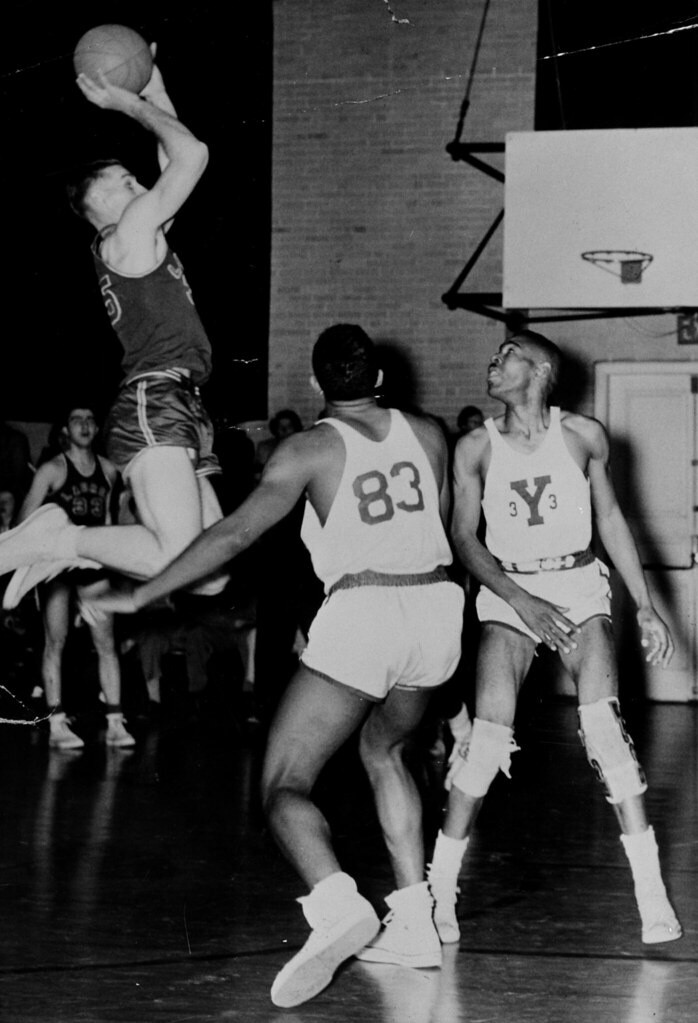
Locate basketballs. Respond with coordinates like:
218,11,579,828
74,20,153,93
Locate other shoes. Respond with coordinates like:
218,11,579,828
136,701,168,720
241,691,262,725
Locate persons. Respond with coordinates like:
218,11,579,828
0,40,231,610
19,405,136,750
425,330,684,944
458,406,483,432
1,408,327,731
80,323,465,1008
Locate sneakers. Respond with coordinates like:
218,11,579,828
353,882,444,968
0,502,83,576
2,557,102,610
106,713,135,745
47,712,85,748
271,872,380,1008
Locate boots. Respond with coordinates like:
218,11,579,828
425,830,471,943
618,825,682,943
